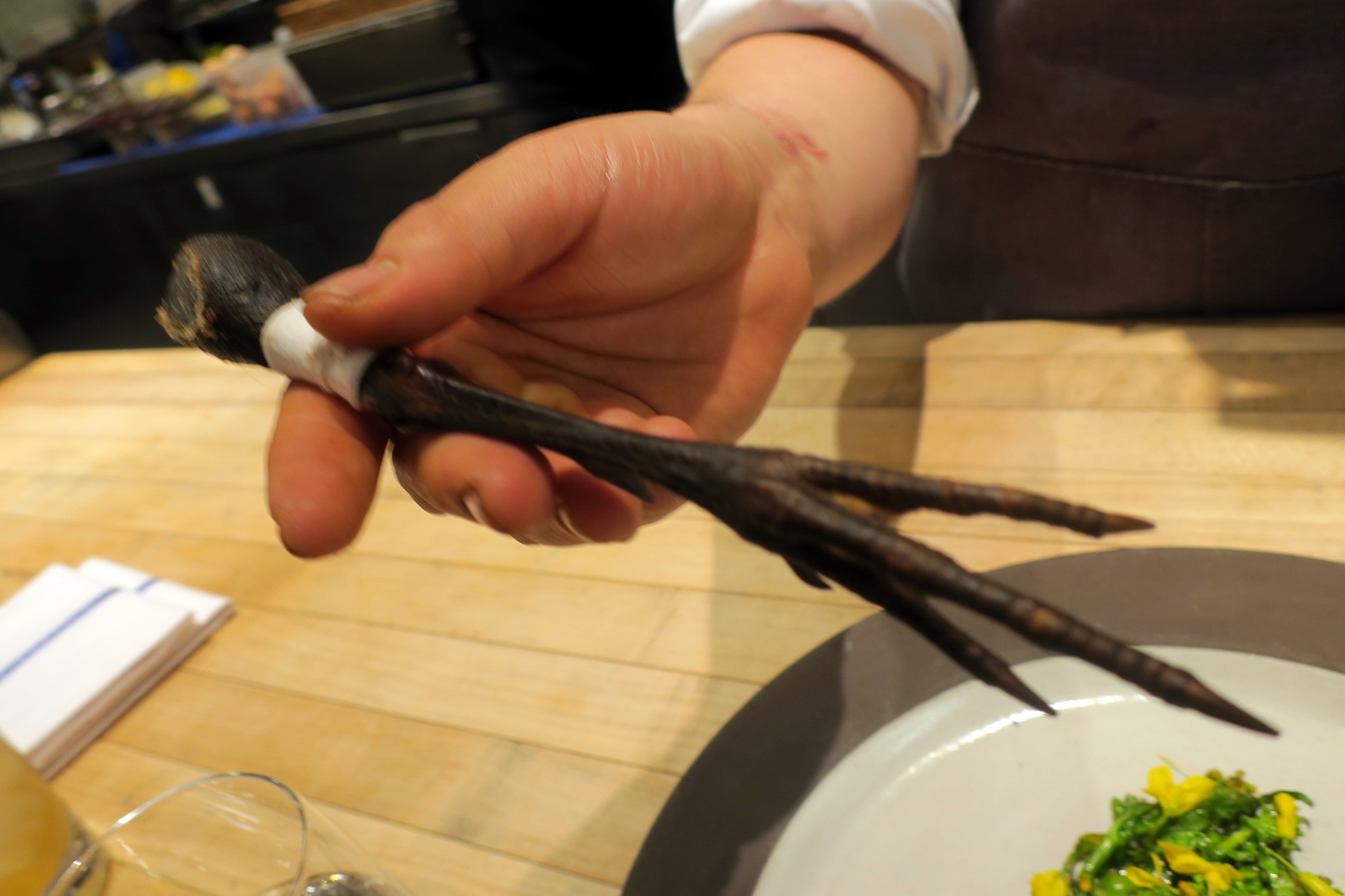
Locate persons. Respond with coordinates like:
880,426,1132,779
266,0,1345,558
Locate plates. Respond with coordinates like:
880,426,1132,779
621,547,1345,896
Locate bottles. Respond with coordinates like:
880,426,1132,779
39,92,75,122
10,77,37,113
81,67,123,100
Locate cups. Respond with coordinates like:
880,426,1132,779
0,735,108,896
45,772,410,896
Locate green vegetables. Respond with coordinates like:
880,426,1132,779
1031,766,1344,896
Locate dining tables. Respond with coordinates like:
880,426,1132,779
0,322,1345,896
